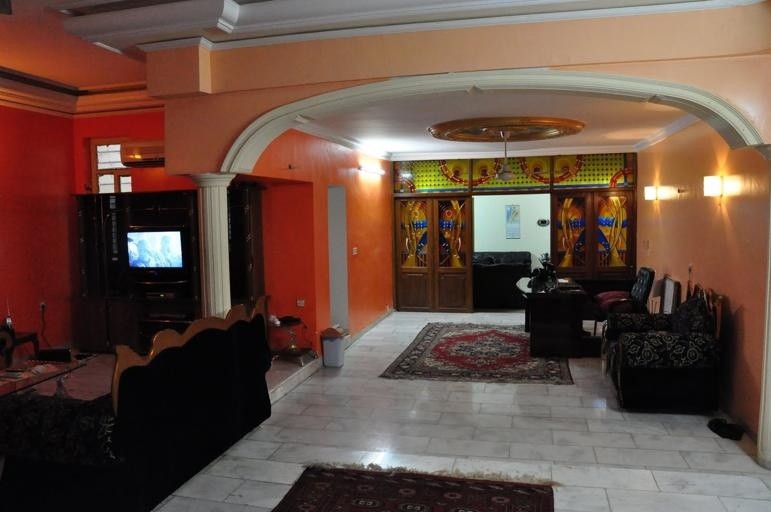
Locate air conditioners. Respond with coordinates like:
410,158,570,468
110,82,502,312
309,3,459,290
118,139,164,168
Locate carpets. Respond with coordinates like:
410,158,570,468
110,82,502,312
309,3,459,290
272,459,557,512
376,322,575,386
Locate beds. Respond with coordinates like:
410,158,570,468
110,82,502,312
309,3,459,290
0,293,275,512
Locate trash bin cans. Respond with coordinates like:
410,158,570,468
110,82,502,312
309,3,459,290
320,324,346,368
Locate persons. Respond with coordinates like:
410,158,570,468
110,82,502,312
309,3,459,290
128,236,176,267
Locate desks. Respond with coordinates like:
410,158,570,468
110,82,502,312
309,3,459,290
514,276,587,359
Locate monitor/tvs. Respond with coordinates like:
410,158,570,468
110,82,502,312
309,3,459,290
124,224,184,281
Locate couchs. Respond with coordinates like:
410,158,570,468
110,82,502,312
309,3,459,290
472,250,532,311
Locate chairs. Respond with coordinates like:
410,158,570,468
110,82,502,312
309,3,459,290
591,266,722,414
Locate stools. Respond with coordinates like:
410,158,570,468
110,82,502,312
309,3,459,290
271,316,318,366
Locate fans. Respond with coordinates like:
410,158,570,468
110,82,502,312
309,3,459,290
475,129,551,184
